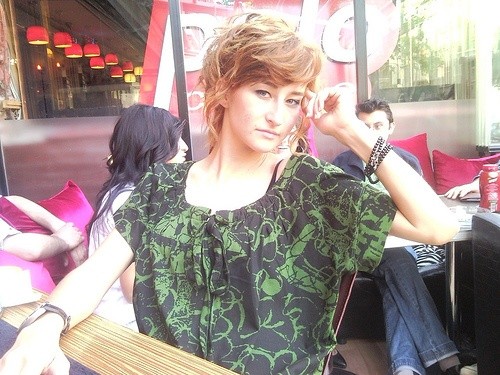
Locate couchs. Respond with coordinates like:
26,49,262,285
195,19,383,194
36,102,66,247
0,98,478,343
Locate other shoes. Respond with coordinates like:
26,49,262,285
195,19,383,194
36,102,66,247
333,350,347,368
446,363,464,375
331,369,355,375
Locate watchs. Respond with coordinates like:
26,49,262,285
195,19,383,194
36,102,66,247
16,301,71,338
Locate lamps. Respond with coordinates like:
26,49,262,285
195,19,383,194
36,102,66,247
54,10,143,83
25,0,49,46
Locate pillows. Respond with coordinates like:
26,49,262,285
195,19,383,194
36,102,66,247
390,132,433,186
433,150,500,195
37,179,94,269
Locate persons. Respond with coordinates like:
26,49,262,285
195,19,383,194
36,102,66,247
1,13,460,375
445,170,481,200
83,103,189,334
0,192,87,269
331,99,464,375
292,136,349,369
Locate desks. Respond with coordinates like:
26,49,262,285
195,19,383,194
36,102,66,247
384,203,493,375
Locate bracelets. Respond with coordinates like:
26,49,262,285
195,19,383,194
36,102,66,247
364,137,394,183
